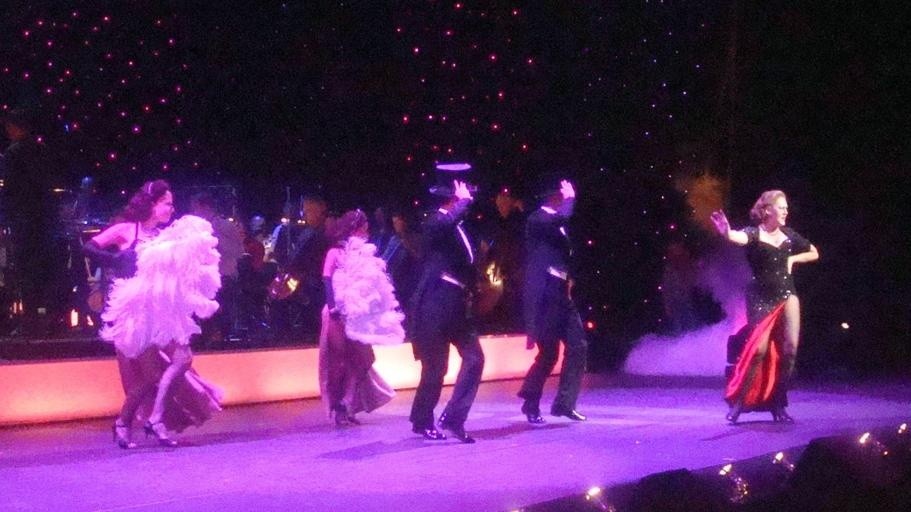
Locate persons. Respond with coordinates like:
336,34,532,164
663,240,705,337
517,177,589,424
403,160,485,443
316,208,396,426
1,110,532,356
710,189,819,424
83,179,223,449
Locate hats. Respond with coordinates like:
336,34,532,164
427,161,480,199
532,172,563,200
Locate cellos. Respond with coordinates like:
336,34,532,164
472,195,522,316
269,210,328,306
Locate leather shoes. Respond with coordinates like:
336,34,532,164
724,410,742,426
436,409,477,444
520,399,548,424
411,421,448,441
328,401,351,427
549,407,587,422
769,408,794,425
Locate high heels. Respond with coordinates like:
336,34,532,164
348,415,362,425
111,420,139,450
142,418,178,448
